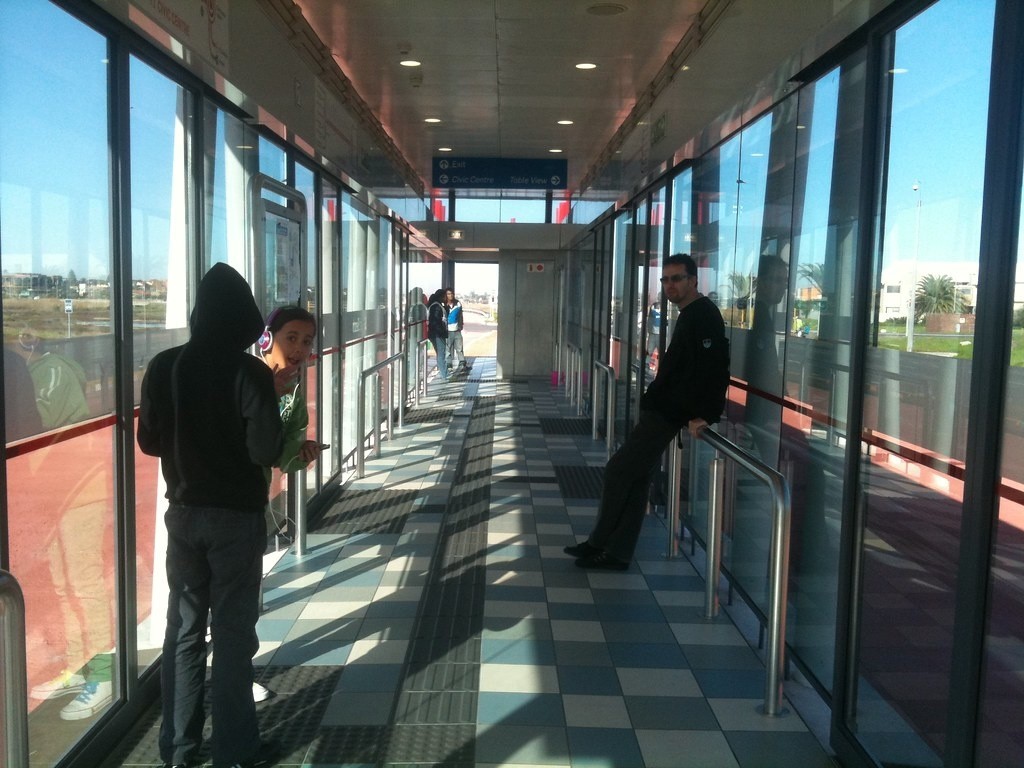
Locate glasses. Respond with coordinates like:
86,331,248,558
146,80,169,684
660,274,694,284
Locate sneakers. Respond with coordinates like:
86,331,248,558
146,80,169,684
60,680,112,720
30,669,87,699
564,541,604,557
252,682,270,702
230,739,281,768
578,553,629,571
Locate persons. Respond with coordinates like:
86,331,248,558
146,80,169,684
244,304,330,703
3,311,126,719
644,292,668,373
428,288,473,381
136,262,281,768
726,255,826,575
562,254,731,573
405,286,429,343
708,292,719,305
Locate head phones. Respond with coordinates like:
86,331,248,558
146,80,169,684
258,307,296,351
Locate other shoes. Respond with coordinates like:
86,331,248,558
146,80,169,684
448,368,454,374
459,363,472,372
